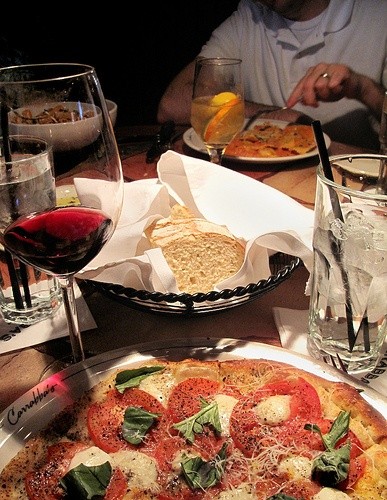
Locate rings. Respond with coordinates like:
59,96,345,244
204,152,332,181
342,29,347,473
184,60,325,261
319,73,331,79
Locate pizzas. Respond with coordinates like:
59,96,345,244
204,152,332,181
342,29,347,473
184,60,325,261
5,359,387,496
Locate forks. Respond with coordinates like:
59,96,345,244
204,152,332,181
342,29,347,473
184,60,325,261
152,121,190,164
241,95,305,134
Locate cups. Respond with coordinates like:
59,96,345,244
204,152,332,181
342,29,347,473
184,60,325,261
306,152,387,376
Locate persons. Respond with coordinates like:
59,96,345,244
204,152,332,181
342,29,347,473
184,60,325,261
159,0,387,151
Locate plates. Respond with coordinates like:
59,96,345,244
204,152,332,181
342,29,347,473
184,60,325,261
1,337,384,499
184,117,331,163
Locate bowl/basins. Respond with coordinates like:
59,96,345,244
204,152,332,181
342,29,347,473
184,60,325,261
4,100,121,152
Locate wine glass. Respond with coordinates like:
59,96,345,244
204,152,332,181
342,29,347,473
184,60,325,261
193,56,244,163
0,136,58,325
0,65,124,371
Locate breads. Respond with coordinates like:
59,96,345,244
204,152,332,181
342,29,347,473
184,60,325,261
143,202,246,295
